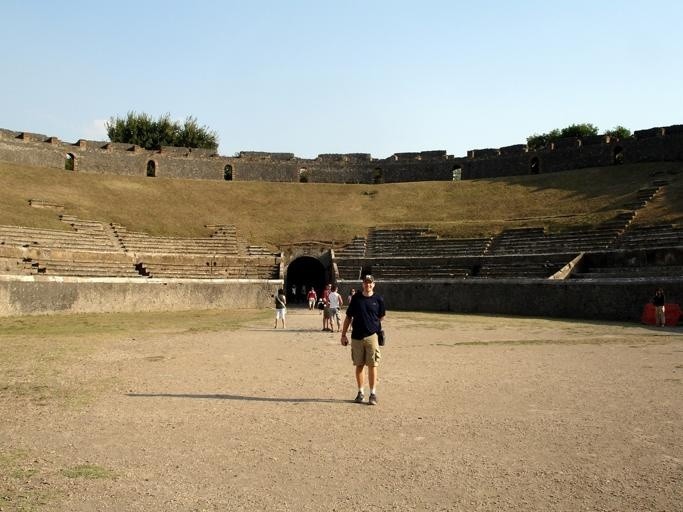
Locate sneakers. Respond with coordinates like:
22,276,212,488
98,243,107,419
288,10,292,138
331,329,334,332
369,393,377,405
275,326,285,328
355,392,365,401
337,329,340,332
323,328,331,331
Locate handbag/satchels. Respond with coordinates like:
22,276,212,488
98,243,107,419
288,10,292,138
378,322,386,347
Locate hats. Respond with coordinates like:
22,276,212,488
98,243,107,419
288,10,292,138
363,275,374,283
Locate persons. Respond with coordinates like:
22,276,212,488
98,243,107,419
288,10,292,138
275,289,286,328
653,288,665,328
341,274,385,405
347,288,356,331
291,283,343,332
